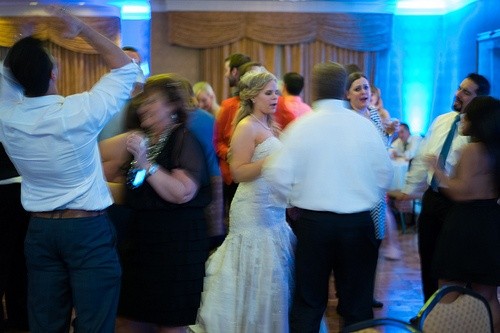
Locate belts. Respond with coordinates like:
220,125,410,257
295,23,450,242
30,210,107,218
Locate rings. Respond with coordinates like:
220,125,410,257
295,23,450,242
56,7,65,18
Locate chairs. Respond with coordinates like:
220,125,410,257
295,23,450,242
390,158,416,233
343,283,494,333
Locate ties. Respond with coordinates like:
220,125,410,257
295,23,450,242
430,114,460,191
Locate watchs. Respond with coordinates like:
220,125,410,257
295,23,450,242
144,163,161,180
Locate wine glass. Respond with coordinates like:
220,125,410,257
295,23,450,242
383,118,397,149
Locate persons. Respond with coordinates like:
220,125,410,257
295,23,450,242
214,62,296,239
193,81,220,114
98,73,213,333
368,84,398,239
196,66,297,333
0,6,146,331
263,61,407,333
399,73,492,324
422,95,500,332
282,71,312,117
224,53,251,98
184,81,225,249
98,47,142,133
392,122,419,160
344,62,416,261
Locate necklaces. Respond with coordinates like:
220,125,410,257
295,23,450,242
250,112,273,132
